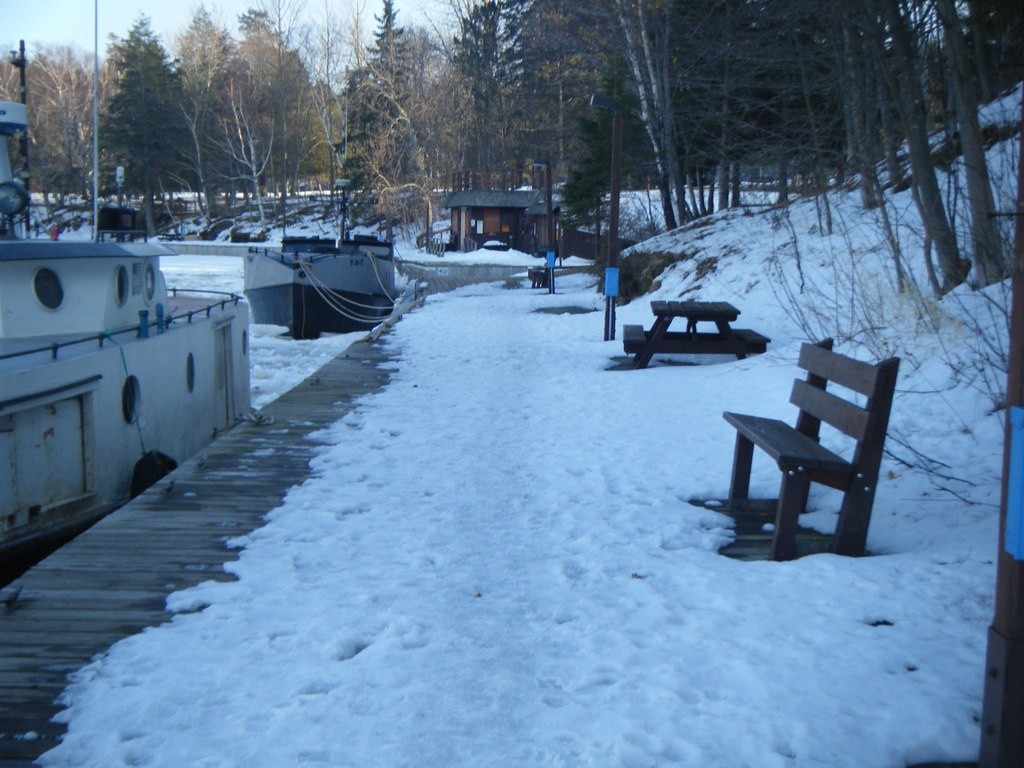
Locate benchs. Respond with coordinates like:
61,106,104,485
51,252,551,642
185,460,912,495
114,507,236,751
732,328,771,354
622,324,645,354
723,336,902,560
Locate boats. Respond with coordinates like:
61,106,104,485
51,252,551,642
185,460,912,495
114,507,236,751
241,175,398,340
0,2,252,570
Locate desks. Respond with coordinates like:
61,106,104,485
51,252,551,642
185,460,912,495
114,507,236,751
630,300,747,370
